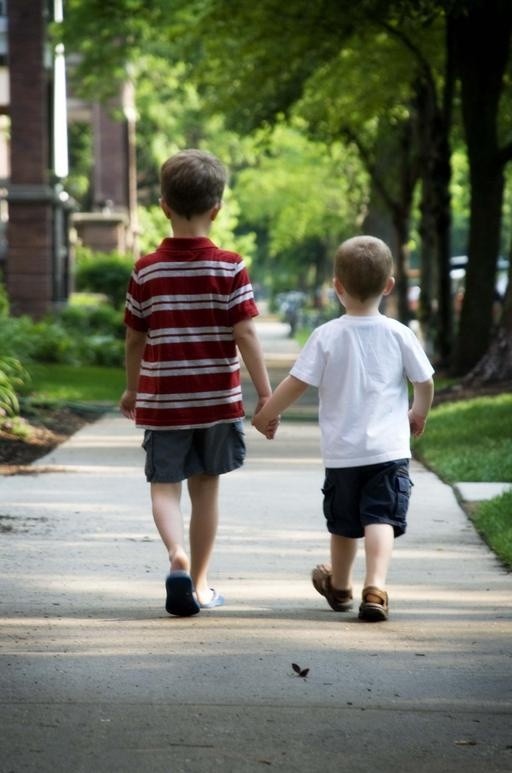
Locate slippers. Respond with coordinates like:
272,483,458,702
200,587,224,608
164,569,201,615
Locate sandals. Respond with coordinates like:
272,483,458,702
357,586,390,620
311,563,354,611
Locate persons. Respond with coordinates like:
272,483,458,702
255,234,436,621
119,148,282,613
